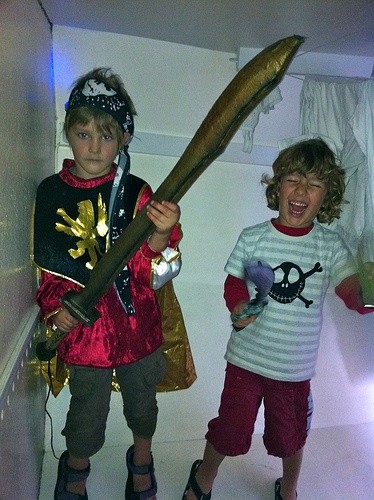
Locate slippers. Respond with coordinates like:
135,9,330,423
275,478,297,500
182,460,212,500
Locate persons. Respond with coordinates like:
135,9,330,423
29,68,197,500
183,137,374,500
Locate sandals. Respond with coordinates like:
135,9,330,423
125,444,157,500
54,450,91,500
